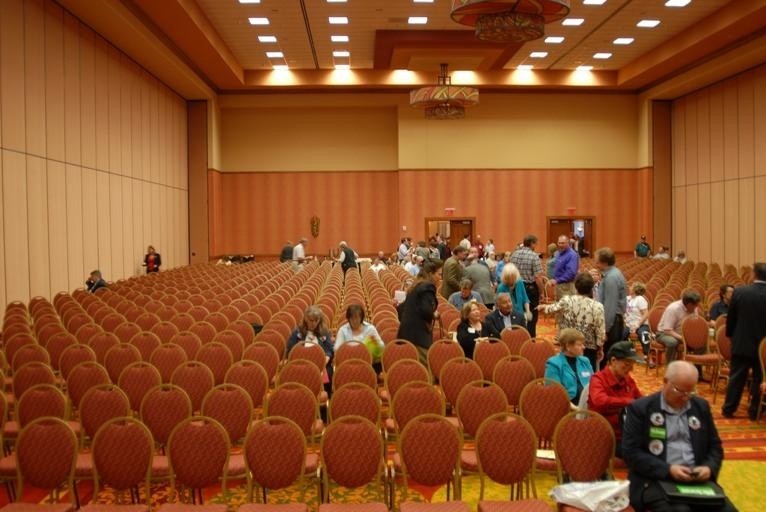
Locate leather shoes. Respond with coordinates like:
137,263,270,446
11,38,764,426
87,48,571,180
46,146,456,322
721,410,757,421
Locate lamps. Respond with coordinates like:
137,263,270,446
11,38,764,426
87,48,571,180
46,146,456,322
410,63,480,119
449,0,571,43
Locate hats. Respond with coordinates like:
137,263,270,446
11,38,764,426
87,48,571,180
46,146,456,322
607,340,647,366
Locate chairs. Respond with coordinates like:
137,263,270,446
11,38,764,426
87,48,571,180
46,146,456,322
0,258,766,512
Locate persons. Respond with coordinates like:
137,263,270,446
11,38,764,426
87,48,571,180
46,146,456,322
216,255,231,266
142,246,161,273
86,271,107,293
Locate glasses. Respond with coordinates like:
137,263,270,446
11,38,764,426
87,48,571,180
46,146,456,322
668,381,699,399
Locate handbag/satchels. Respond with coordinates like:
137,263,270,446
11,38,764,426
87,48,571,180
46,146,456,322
548,480,632,512
